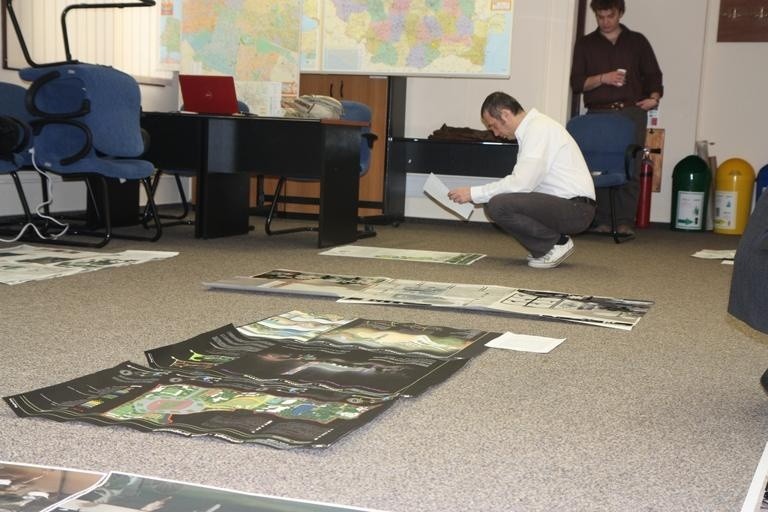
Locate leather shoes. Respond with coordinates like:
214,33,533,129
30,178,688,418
589,224,612,236
614,224,635,243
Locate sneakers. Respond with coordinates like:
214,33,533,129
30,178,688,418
525,254,535,261
527,236,576,269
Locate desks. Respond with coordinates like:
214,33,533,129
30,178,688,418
88,112,370,247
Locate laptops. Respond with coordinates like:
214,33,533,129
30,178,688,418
178,74,256,115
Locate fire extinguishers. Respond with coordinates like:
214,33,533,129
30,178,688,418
638,147,660,228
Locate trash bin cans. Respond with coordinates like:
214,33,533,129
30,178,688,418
712,159,754,237
755,165,768,201
671,155,709,232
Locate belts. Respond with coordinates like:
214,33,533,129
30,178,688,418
594,101,627,109
577,197,596,205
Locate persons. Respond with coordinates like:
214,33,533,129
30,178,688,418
570,0,664,240
447,91,596,269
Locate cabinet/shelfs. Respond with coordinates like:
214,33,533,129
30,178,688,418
395,136,519,179
192,73,406,228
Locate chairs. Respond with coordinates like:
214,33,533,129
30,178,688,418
567,112,641,243
140,100,250,227
265,101,377,239
0,0,161,249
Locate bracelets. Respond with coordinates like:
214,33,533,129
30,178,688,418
599,72,603,85
649,97,660,108
